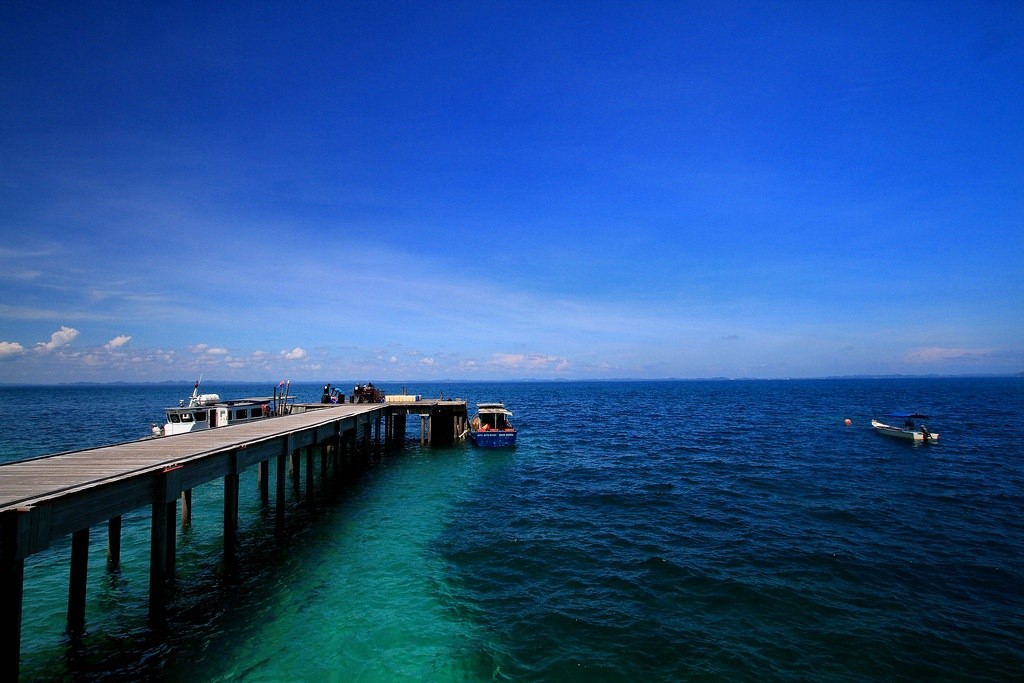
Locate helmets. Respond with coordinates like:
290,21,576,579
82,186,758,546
356,382,359,386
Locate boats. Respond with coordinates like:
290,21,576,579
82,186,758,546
872,411,939,442
145,371,298,438
471,399,518,448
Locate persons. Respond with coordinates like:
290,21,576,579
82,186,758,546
152,423,164,437
472,413,482,430
324,383,342,404
353,383,385,404
262,403,271,417
